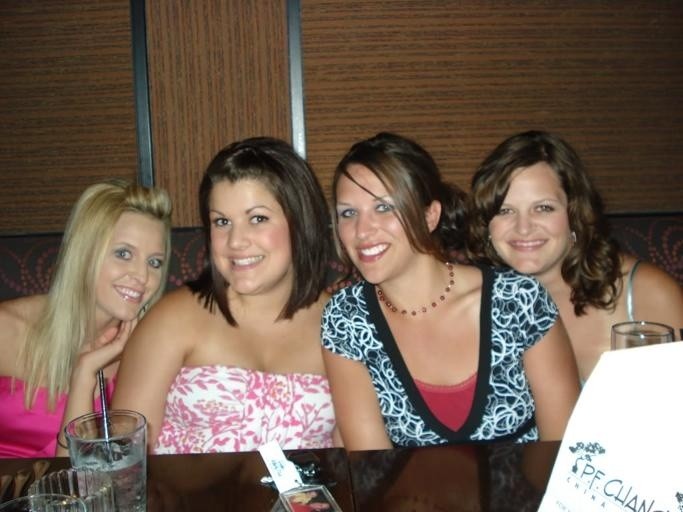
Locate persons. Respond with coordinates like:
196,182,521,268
464,128,683,382
114,133,344,455
0,178,171,459
320,131,581,452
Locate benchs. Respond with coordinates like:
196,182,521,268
1,208,683,305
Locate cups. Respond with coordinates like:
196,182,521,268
28,465,116,512
64,410,150,512
608,319,676,349
0,493,86,512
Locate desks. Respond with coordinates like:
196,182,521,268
0,434,356,511
347,437,561,510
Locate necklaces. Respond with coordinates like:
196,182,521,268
377,262,454,316
